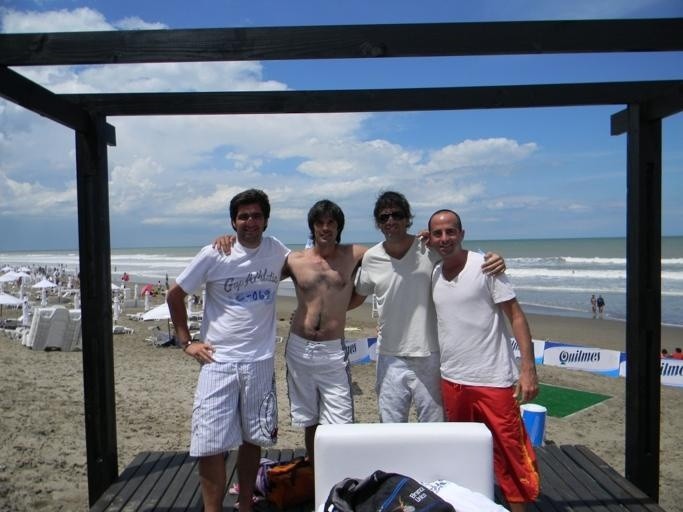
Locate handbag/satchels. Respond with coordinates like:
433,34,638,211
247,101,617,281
255,456,314,509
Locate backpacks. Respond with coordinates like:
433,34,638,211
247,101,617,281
324,470,456,512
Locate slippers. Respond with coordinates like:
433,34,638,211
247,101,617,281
229,483,260,509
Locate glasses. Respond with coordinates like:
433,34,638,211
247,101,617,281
377,211,406,224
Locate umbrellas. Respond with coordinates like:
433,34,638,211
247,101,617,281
31,278,58,303
0,291,26,305
0,271,20,282
141,301,194,340
18,266,31,272
18,272,31,299
1,266,14,271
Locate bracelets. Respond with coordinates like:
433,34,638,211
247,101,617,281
184,339,193,351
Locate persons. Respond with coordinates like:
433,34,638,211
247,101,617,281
661,349,672,358
427,209,543,504
122,272,129,289
672,348,683,359
166,188,292,512
211,197,430,465
54,268,60,283
345,189,507,422
157,280,161,291
591,295,597,314
597,294,605,314
